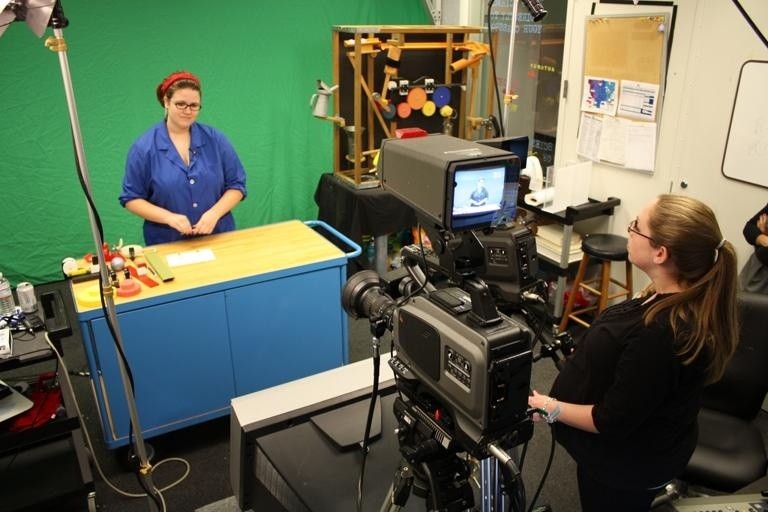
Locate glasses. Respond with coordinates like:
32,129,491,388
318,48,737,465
626,219,653,243
169,99,201,112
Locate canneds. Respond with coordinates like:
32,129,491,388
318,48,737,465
16,282,38,314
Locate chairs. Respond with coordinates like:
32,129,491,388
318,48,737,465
678,287,767,500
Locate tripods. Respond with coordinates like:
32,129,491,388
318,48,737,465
378,425,475,512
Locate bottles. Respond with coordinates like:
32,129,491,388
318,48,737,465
0,273,17,317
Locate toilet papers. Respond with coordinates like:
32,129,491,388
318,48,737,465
524,188,555,207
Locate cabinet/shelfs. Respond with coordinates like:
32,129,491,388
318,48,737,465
0,287,107,512
62,214,367,467
511,183,624,344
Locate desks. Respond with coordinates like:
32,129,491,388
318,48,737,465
312,169,423,302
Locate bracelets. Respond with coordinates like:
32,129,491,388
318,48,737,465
541,397,556,416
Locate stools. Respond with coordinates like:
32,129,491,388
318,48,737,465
554,231,635,338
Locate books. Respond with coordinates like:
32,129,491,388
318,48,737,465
535,223,584,264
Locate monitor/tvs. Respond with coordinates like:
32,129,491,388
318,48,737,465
451,160,508,219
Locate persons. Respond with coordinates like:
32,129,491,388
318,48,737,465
737,202,767,293
527,194,739,512
117,72,248,246
468,178,489,206
